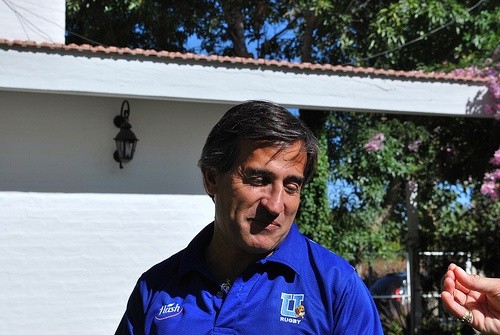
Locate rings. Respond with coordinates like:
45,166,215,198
461,310,472,326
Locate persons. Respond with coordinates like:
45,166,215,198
441,263,500,335
114,101,384,335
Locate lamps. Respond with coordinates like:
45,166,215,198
113,100,139,168
